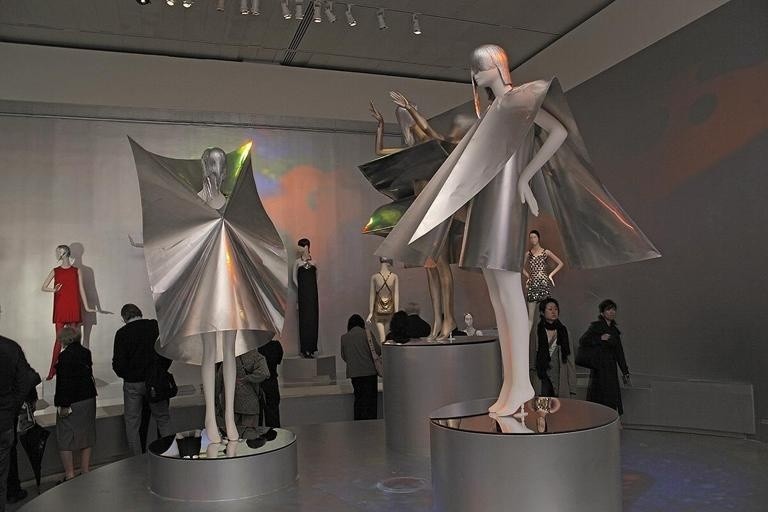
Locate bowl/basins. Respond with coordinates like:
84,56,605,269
300,255,312,269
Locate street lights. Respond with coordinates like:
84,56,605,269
145,373,178,403
574,344,602,369
365,329,383,377
258,389,268,410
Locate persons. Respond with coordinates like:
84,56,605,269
525,396,561,434
463,311,482,336
216,350,271,427
122,130,289,444
360,89,479,342
529,298,578,398
340,313,382,421
111,303,177,458
366,256,399,343
372,43,664,416
574,299,633,415
41,244,96,381
291,237,321,358
1,333,41,511
521,229,564,351
257,339,284,428
54,326,98,485
238,427,278,449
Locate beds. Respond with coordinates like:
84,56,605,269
19,422,52,494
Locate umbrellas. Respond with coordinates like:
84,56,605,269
6,490,27,504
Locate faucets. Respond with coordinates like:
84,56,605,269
56,477,66,485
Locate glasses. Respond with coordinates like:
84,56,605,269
136,0,421,35
